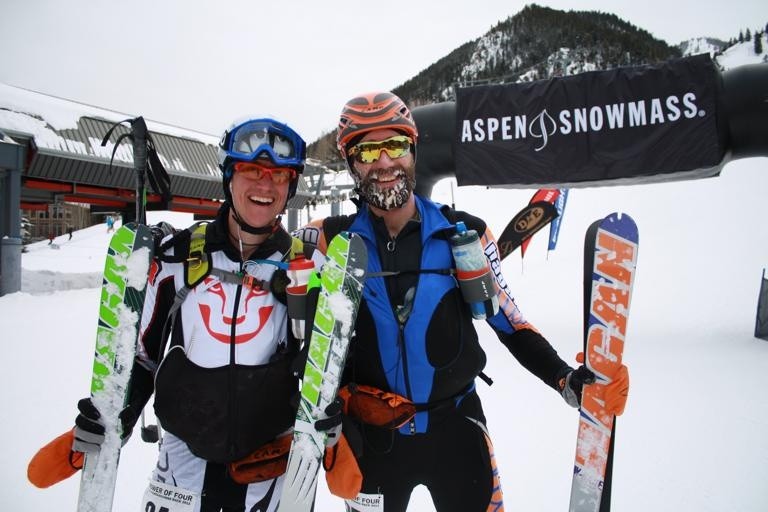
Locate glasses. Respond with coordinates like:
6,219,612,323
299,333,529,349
344,136,414,165
233,161,298,185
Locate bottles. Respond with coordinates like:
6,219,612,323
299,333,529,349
450,221,501,318
286,252,318,339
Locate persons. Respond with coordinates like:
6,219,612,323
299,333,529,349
68,110,345,512
105,214,118,234
67,223,74,241
45,230,55,246
284,90,631,511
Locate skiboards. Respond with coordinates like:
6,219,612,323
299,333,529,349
569,214,638,512
79,221,367,512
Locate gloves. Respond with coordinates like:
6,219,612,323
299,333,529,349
314,394,346,449
69,394,136,456
558,349,630,419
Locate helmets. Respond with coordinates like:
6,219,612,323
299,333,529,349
217,117,307,176
336,91,419,160
